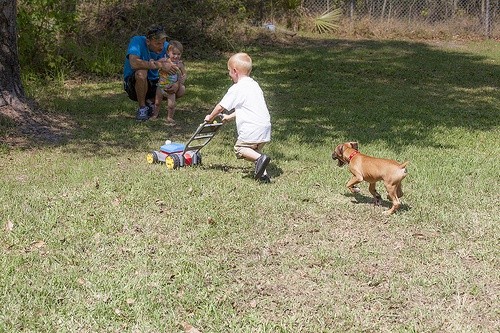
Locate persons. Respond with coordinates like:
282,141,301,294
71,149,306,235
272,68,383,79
150,41,186,122
204,52,272,184
123,24,186,121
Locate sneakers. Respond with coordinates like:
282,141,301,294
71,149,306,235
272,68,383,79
144,99,154,116
137,106,149,122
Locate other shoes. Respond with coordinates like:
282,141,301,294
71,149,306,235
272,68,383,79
252,153,270,181
242,174,271,183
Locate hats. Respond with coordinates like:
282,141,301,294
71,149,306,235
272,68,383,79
147,26,169,41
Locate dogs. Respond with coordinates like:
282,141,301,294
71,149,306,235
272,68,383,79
331,142,410,215
